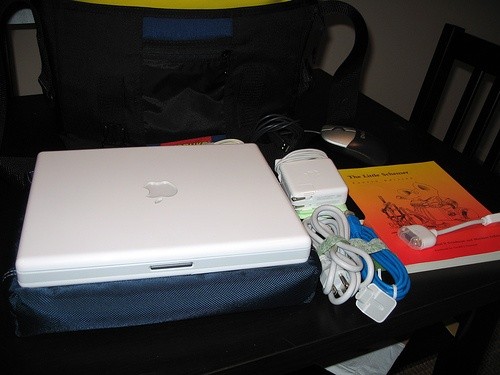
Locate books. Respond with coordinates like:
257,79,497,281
337,161,500,275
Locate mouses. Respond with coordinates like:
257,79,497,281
320,124,389,166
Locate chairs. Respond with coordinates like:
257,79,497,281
408,23,500,178
0,0,50,98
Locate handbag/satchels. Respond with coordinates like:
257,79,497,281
7,249,322,338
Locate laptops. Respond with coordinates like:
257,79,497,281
15,141,312,288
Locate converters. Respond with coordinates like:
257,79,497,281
278,157,349,211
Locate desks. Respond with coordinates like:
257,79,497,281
0,68,500,373
